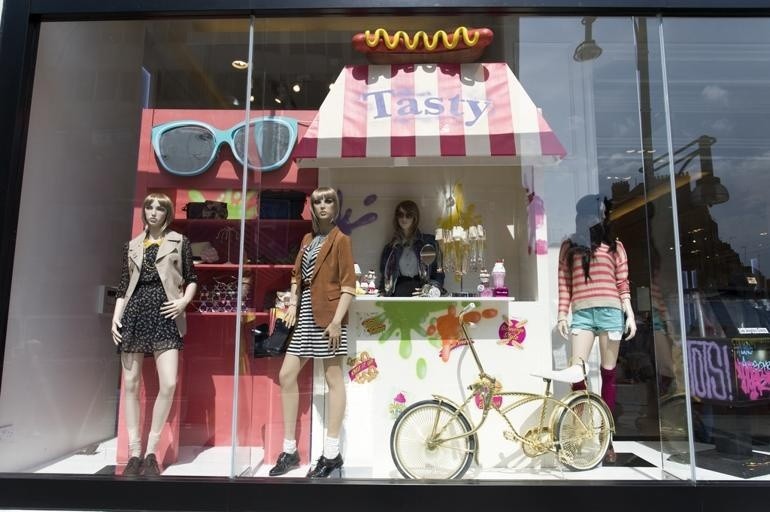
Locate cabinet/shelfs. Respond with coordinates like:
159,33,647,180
112,108,319,476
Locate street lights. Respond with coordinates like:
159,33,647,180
575,20,730,209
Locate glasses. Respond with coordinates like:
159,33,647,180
149,115,300,178
396,211,413,220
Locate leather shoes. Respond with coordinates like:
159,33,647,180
601,441,615,464
122,458,143,476
141,454,160,476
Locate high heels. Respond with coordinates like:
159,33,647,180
307,454,343,479
271,449,300,477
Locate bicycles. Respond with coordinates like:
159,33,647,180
390,302,615,480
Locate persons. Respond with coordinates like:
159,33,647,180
558,194,637,463
378,200,447,296
110,192,199,479
269,186,356,479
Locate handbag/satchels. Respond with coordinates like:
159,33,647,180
254,187,307,219
263,318,294,354
181,198,230,220
196,275,254,315
